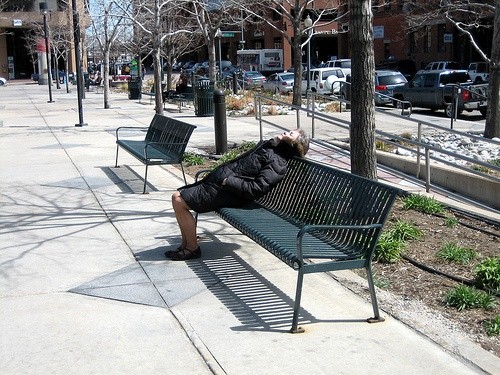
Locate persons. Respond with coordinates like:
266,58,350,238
165,129,309,261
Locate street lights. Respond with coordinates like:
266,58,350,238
216,28,223,81
305,13,315,139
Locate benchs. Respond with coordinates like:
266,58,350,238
115,115,197,195
139,83,195,113
195,155,403,333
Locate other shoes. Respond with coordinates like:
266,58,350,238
172,246,202,261
164,246,183,258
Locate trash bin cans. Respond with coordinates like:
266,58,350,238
128,80,142,100
194,81,215,117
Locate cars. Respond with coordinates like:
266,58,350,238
226,71,266,90
261,72,294,95
31,68,74,81
287,63,316,73
149,57,233,87
338,70,408,107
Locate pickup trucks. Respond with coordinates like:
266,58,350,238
392,69,489,118
467,62,491,84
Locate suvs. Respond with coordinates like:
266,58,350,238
298,67,347,96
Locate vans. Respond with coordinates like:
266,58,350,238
322,59,351,77
376,58,417,81
425,62,461,70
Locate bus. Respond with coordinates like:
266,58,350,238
237,49,284,75
